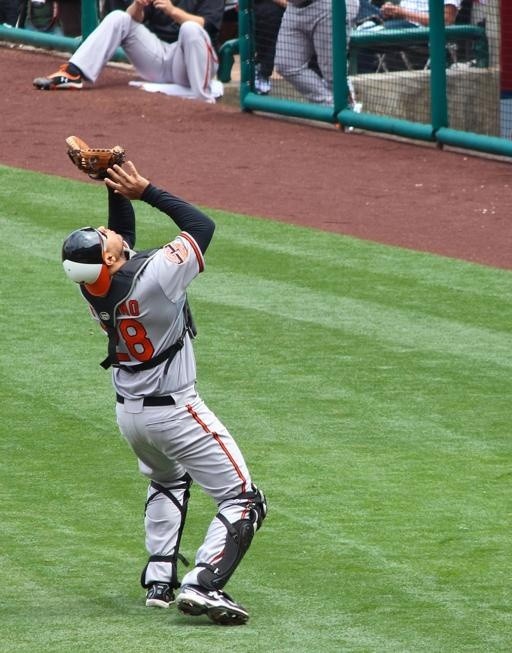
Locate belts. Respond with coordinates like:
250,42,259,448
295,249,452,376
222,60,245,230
111,392,177,407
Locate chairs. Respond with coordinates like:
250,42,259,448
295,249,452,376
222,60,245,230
371,0,474,71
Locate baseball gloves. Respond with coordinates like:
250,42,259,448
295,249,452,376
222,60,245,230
65,136,125,180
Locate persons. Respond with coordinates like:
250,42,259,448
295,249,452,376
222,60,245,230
18,1,462,133
61,136,267,626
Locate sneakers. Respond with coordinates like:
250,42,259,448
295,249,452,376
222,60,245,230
252,65,271,95
143,582,177,609
32,63,82,91
174,583,250,626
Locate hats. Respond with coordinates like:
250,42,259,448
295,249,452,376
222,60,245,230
61,225,111,297
